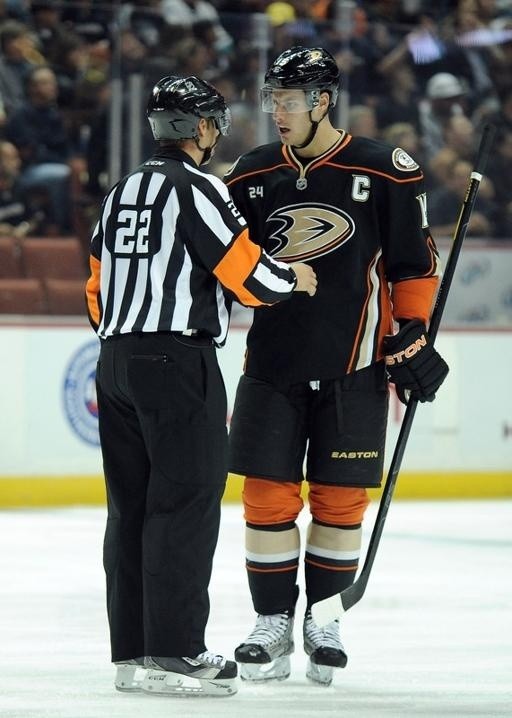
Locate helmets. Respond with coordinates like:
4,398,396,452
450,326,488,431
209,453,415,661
146,75,230,140
427,72,463,99
260,46,341,113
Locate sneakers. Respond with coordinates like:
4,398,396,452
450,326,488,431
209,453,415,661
302,610,348,668
113,651,237,679
234,610,294,662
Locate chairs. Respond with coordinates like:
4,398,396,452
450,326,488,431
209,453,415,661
0,236,48,315
19,237,92,316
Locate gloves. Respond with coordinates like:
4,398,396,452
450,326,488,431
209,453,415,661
380,317,450,404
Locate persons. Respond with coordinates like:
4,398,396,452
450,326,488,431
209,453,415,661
2,2,511,242
221,46,450,670
82,75,319,683
